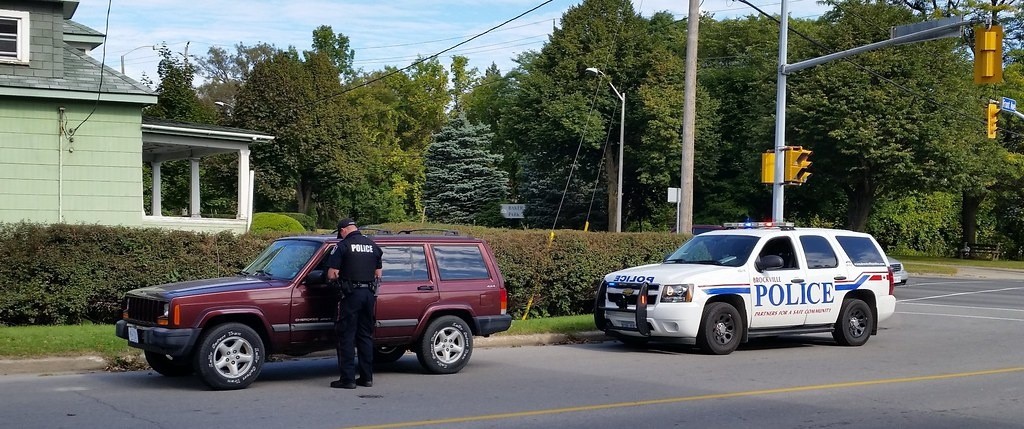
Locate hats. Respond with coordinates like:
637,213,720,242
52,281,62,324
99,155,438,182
337,218,354,239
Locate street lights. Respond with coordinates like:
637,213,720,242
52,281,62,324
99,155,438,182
586,67,626,233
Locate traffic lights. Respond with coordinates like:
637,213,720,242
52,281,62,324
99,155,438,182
802,149,813,183
988,104,1001,139
785,149,806,184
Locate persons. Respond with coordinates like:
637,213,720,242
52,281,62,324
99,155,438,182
328,219,384,389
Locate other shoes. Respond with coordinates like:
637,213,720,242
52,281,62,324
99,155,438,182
330,379,357,389
355,378,373,387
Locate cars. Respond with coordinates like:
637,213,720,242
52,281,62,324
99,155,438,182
805,250,908,287
670,225,725,235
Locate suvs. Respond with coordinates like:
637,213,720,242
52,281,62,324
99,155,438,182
115,227,513,390
593,222,896,356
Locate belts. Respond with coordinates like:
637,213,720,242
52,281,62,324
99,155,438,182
353,283,369,288
